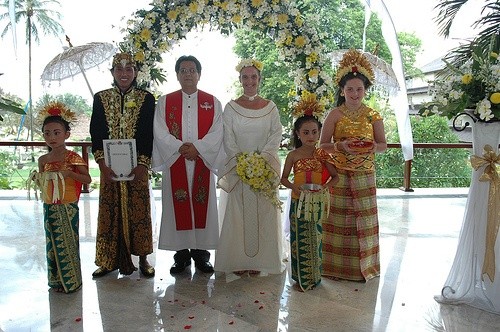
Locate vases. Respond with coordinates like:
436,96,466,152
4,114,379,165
436,122,500,314
462,109,473,126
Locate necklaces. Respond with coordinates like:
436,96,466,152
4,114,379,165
243,93,258,101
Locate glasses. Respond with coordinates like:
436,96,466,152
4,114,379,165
178,68,198,73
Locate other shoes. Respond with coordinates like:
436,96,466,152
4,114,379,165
139,260,155,278
92,266,111,277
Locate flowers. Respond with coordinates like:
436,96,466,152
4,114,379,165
337,63,372,81
236,152,283,213
420,30,500,122
110,1,335,114
36,101,77,124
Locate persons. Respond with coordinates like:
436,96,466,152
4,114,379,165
90,54,157,277
213,59,289,276
28,115,92,294
150,54,225,274
281,114,339,293
319,70,387,283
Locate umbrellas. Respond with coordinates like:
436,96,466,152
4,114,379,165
40,34,115,98
324,43,400,104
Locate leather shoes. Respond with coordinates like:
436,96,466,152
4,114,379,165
195,260,214,273
170,259,191,274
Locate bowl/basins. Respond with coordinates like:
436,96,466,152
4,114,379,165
301,184,323,193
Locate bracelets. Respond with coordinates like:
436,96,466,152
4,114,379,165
372,141,377,154
333,141,339,152
